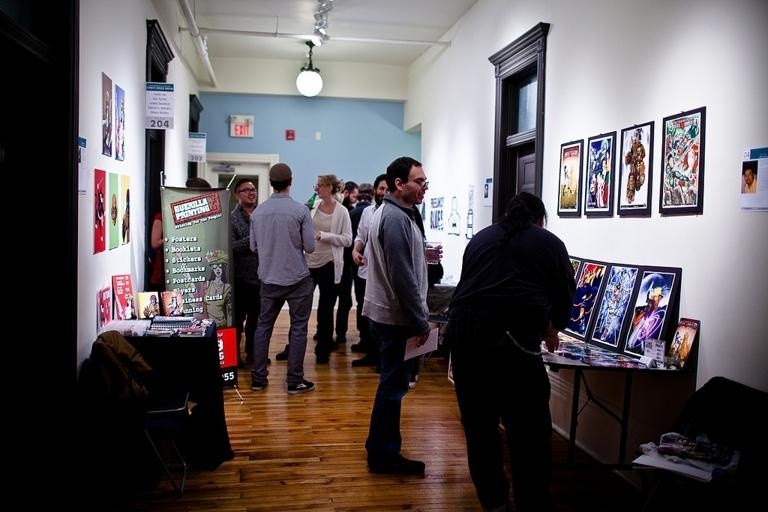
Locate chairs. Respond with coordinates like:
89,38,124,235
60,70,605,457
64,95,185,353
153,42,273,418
588,374,768,509
90,330,192,497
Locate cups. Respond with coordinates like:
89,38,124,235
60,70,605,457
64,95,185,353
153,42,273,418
424,240,442,266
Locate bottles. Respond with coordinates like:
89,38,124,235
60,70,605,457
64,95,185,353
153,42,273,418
305,192,317,208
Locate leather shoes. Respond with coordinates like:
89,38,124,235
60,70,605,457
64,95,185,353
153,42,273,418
352,358,381,366
244,354,271,369
368,453,426,475
351,341,367,352
313,332,340,364
408,374,420,389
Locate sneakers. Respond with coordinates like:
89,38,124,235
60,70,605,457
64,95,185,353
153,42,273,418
287,380,316,396
251,378,268,391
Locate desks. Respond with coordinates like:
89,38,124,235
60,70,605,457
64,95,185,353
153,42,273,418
536,347,695,478
613,454,742,511
115,318,234,472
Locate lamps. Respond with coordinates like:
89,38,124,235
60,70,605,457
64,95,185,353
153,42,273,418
294,38,325,98
310,0,334,48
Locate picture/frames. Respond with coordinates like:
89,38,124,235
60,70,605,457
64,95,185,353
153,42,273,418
560,255,684,364
557,105,708,218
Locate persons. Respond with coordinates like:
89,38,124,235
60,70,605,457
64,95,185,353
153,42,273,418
199,249,232,328
149,213,165,291
230,179,271,369
248,162,315,395
445,191,576,512
302,170,388,368
364,156,443,476
744,168,757,194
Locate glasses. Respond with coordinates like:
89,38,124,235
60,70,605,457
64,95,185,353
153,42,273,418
212,263,223,269
413,180,429,187
239,188,257,193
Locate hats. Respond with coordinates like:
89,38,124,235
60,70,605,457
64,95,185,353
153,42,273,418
269,162,292,182
209,249,229,266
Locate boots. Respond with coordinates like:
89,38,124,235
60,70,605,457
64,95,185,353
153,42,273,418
276,345,290,360
